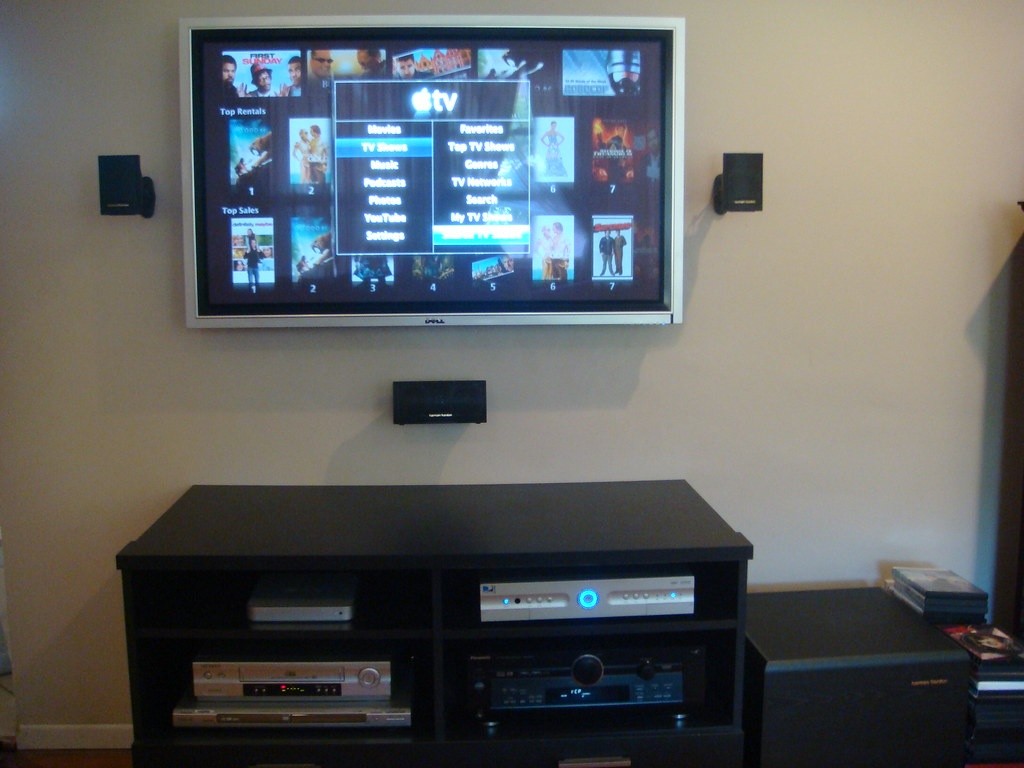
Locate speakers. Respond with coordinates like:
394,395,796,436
723,153,762,211
98,155,144,216
390,379,488,426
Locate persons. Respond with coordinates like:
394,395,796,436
357,42,386,77
238,60,290,98
307,49,335,98
541,120,569,179
293,125,328,184
287,56,302,97
502,255,514,273
232,228,274,296
599,228,627,277
537,221,570,281
222,55,239,98
393,53,434,81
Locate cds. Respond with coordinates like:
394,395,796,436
960,632,1024,653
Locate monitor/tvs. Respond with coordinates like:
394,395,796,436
178,17,683,326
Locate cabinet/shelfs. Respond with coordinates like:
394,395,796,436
115,480,753,768
